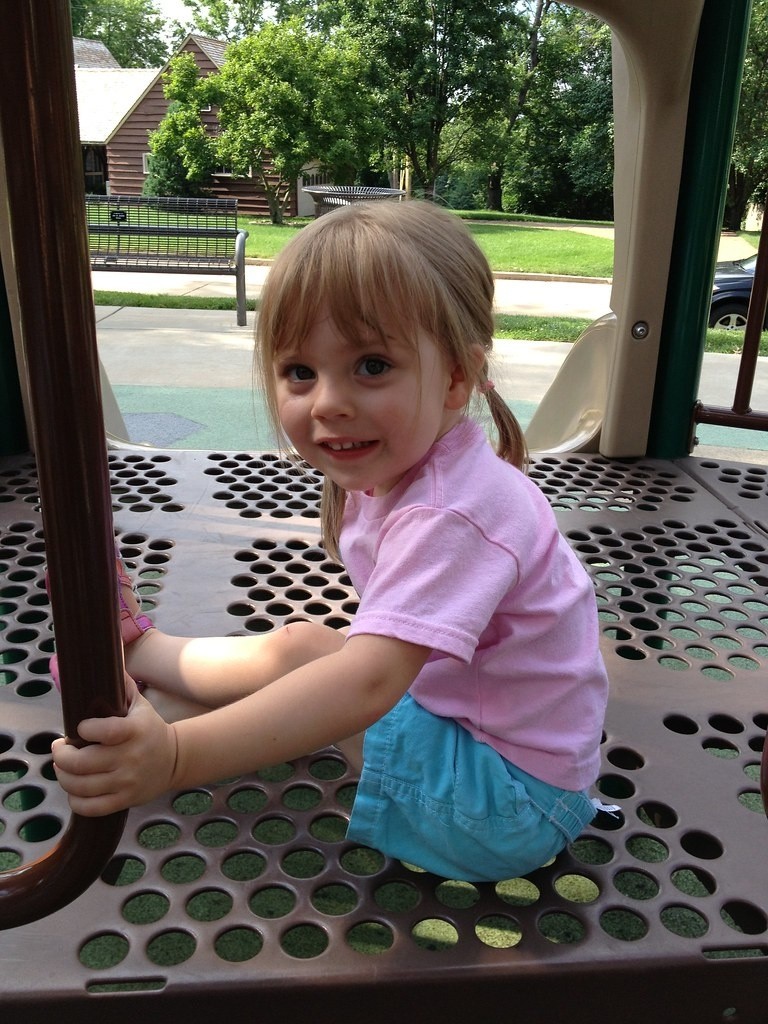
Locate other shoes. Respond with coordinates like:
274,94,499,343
44,540,155,645
49,655,61,690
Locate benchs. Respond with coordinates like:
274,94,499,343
84,193,249,327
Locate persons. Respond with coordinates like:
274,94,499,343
45,198,609,883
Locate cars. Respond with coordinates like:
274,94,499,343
707,253,768,331
